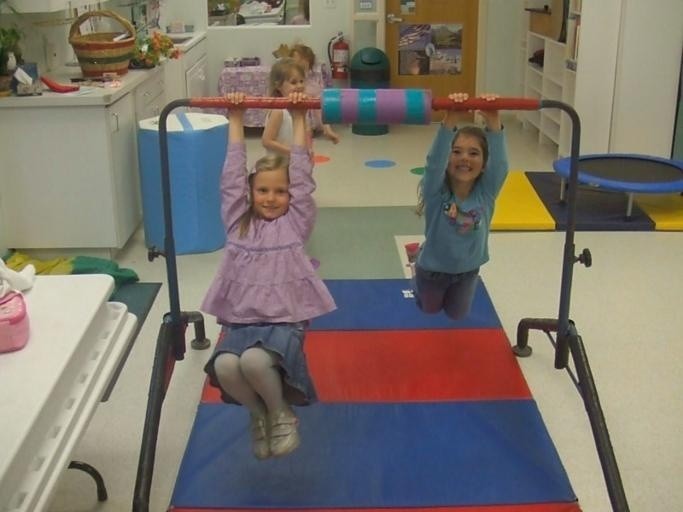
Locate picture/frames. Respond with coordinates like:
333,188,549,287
204,0,313,31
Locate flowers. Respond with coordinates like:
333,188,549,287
133,29,181,69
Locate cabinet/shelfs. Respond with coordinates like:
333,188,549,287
522,0,622,162
165,39,215,104
1,71,165,249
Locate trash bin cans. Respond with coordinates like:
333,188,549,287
350,48,390,135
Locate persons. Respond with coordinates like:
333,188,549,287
204,92,336,459
405,93,509,318
261,44,340,157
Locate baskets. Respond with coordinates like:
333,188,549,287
69,11,135,77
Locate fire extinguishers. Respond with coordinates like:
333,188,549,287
328,32,350,80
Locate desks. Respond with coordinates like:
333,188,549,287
0,269,140,509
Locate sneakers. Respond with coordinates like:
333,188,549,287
250,408,269,460
270,408,301,457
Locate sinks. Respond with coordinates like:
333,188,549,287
170,37,190,44
